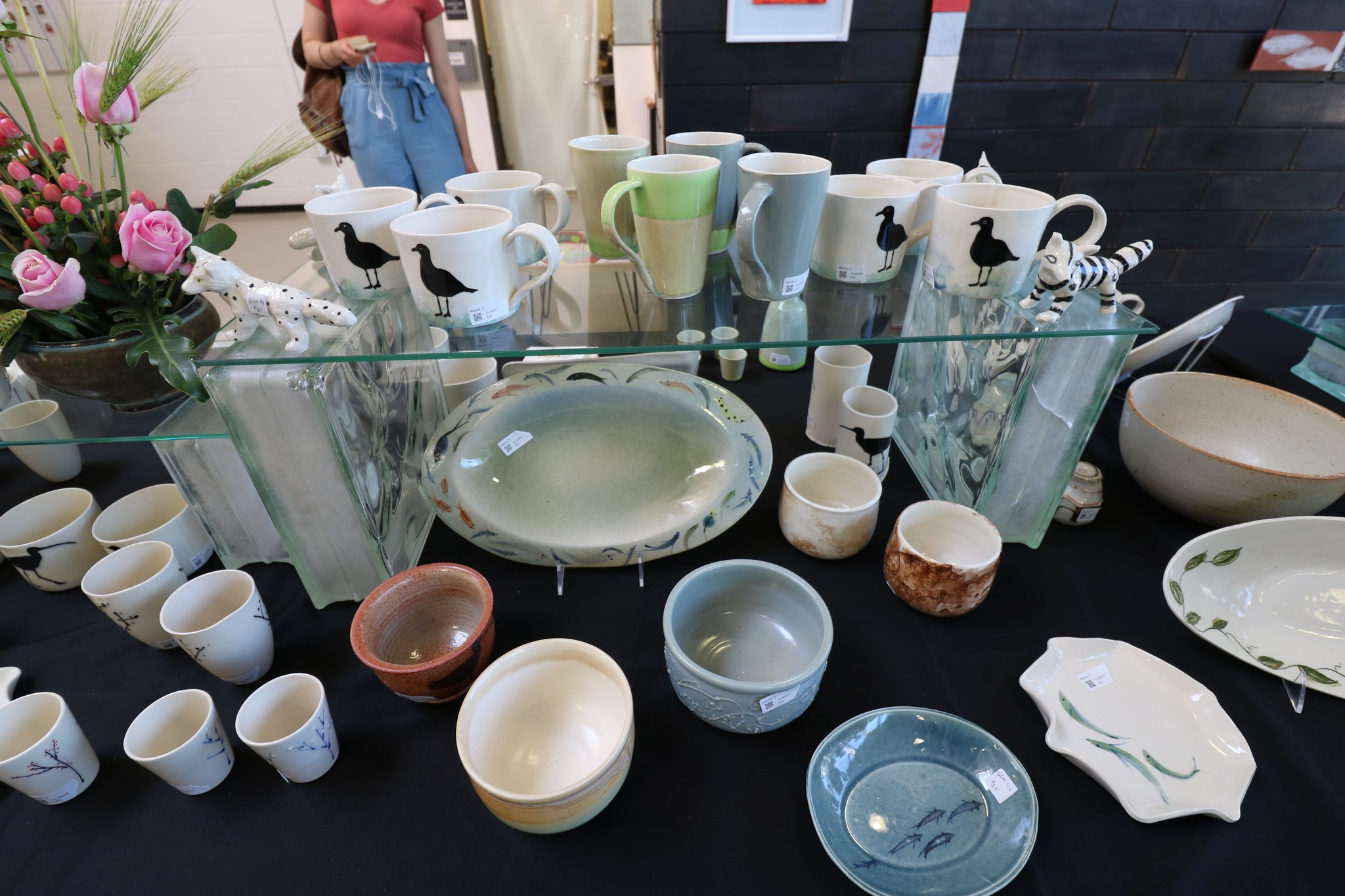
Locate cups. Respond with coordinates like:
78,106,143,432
304,186,459,299
390,204,560,328
445,171,571,267
804,343,899,484
920,182,1107,299
347,563,496,705
778,453,884,557
398,327,499,414
677,326,747,381
885,500,1003,618
0,399,341,807
1115,289,1145,315
567,131,1002,299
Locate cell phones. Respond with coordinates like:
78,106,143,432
353,42,377,54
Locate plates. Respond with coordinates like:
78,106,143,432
1120,294,1245,375
1018,636,1258,825
804,705,1039,896
418,360,774,567
1163,516,1345,699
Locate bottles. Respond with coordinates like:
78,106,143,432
758,296,808,370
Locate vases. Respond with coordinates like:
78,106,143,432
17,293,221,423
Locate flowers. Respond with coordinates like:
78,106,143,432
0,0,352,365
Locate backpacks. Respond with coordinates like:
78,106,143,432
292,0,351,158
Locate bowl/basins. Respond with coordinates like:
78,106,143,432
455,638,636,835
662,559,835,734
1118,370,1345,528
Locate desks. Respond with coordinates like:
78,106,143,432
0,330,1345,896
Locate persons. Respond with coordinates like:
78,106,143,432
301,1,480,208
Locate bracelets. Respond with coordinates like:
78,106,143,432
318,42,336,70
328,41,345,65
